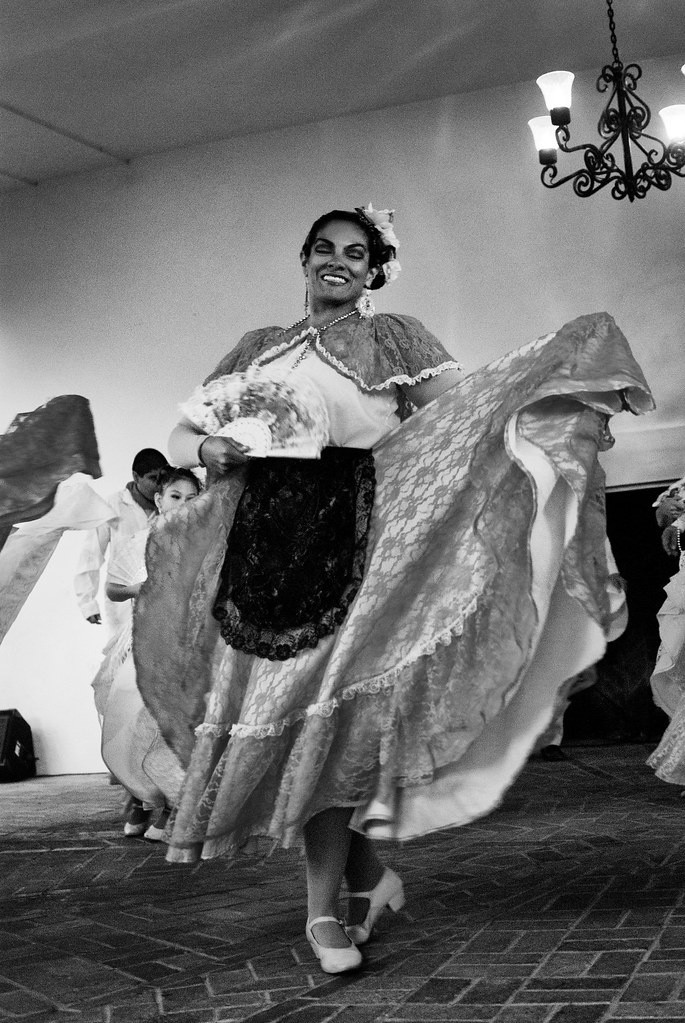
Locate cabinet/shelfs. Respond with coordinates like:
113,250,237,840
561,482,679,745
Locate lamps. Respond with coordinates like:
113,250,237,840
527,0,684,203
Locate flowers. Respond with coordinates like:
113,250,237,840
364,200,400,284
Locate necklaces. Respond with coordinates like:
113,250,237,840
278,308,361,370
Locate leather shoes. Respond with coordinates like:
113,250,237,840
339,866,405,942
124,804,152,837
304,916,362,976
145,809,172,841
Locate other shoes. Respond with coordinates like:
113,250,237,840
541,744,564,761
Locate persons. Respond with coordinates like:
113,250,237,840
644,508,685,798
130,202,655,974
102,467,206,841
78,447,170,655
